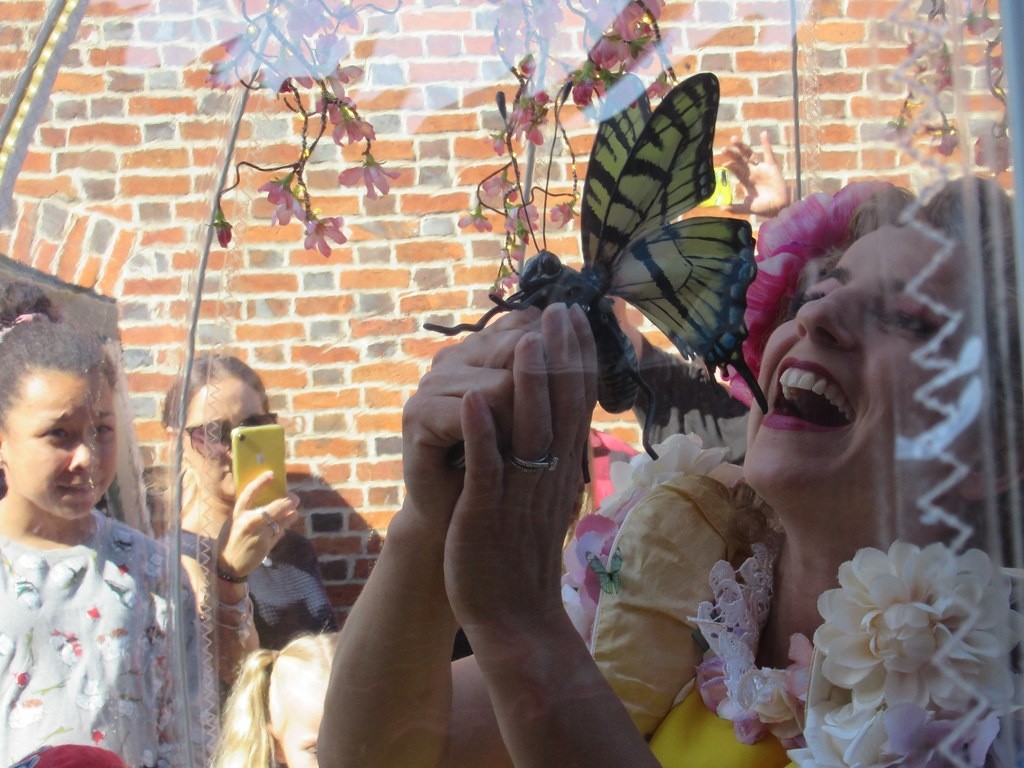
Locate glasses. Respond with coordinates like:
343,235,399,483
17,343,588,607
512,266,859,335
185,413,278,460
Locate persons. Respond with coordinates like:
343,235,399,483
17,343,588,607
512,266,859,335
0,280,220,768
162,354,339,706
211,629,341,768
318,176,1024,768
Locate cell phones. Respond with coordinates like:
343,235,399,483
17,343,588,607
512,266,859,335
231,424,286,508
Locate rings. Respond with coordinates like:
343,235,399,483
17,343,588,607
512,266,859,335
510,449,559,473
259,509,274,526
270,522,279,535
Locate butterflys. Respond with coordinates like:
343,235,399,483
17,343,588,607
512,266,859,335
422,73,769,462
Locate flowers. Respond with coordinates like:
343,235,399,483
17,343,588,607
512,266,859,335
209,0,1024,768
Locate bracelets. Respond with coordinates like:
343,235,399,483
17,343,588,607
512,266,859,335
218,568,250,583
214,584,255,647
